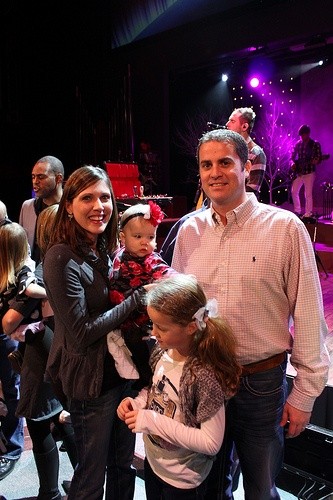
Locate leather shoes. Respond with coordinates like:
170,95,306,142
0,456,20,482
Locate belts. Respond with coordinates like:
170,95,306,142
236,353,288,378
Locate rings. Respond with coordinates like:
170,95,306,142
303,427,305,429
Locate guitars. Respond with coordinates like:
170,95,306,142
287,154,330,182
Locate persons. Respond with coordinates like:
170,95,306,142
290,124,322,221
173,129,330,500
0,156,177,500
116,275,242,499
225,107,266,205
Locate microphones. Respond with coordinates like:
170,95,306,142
207,122,227,129
